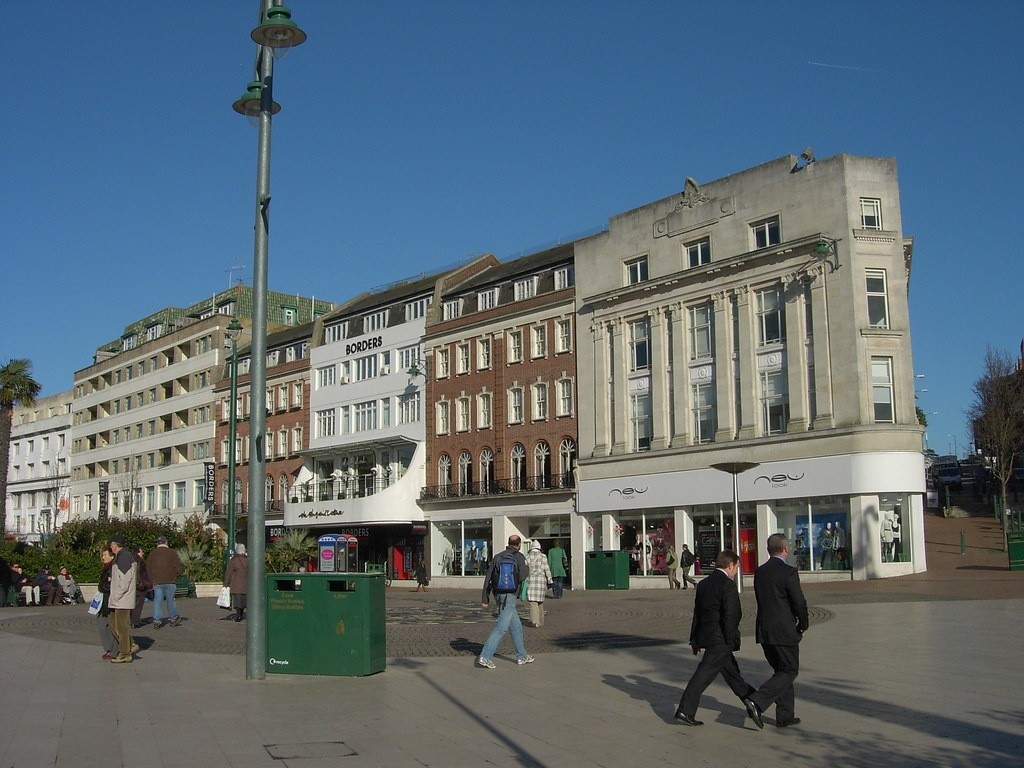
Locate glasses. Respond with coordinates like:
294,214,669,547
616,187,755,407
13,566,19,568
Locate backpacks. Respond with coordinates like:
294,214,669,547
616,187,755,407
685,552,696,566
494,552,519,593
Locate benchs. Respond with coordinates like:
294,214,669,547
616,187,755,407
172,574,196,597
6,580,77,608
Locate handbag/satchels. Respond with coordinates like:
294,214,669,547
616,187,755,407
86,589,104,616
665,551,675,566
520,579,528,602
561,548,569,572
216,585,231,608
423,578,429,586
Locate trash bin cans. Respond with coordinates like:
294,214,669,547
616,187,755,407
367,564,385,573
585,550,630,590
266,572,387,676
1007,530,1024,571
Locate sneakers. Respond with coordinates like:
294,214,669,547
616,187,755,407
110,652,133,663
130,643,140,655
516,654,536,665
477,655,497,669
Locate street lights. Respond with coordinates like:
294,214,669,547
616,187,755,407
224,312,245,565
231,0,309,682
947,434,956,455
709,461,761,595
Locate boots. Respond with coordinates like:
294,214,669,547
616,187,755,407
235,608,245,622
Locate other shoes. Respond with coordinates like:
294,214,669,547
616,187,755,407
101,651,115,660
170,619,183,627
153,620,168,629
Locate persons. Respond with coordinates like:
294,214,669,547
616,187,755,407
10,561,41,607
469,541,489,575
743,533,809,729
680,544,698,590
548,539,569,600
224,543,249,623
57,566,77,605
414,560,428,592
524,540,553,628
821,522,846,570
130,536,181,630
880,512,901,562
96,535,139,663
665,544,680,590
674,549,757,726
36,564,63,606
477,535,535,668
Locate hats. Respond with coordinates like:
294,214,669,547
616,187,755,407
157,536,167,544
59,566,65,571
109,535,126,545
41,565,49,570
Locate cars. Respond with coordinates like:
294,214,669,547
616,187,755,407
1008,468,1024,491
12,531,43,549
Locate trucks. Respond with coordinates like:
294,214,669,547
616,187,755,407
932,455,963,492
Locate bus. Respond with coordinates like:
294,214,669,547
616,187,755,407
958,459,981,489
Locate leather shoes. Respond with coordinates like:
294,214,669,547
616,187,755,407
742,697,769,731
775,717,800,726
674,710,704,726
